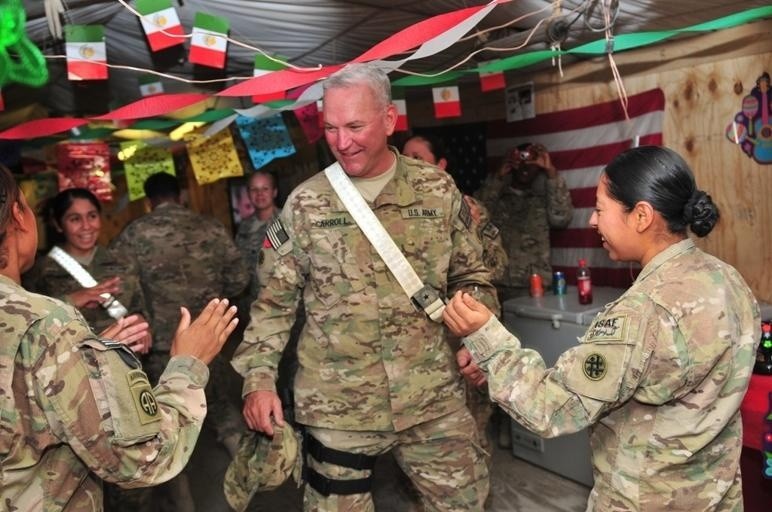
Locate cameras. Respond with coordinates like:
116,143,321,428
512,143,536,162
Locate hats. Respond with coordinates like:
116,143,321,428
220,414,299,512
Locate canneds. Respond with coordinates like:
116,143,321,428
553,271,566,296
529,273,544,297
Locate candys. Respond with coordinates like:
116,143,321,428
726,122,758,146
742,95,759,135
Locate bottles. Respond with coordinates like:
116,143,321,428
760,392,772,478
760,324,772,373
576,259,593,305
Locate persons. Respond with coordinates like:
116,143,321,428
230,61,501,512
442,145,762,512
403,135,510,458
473,142,574,449
0,165,241,512
